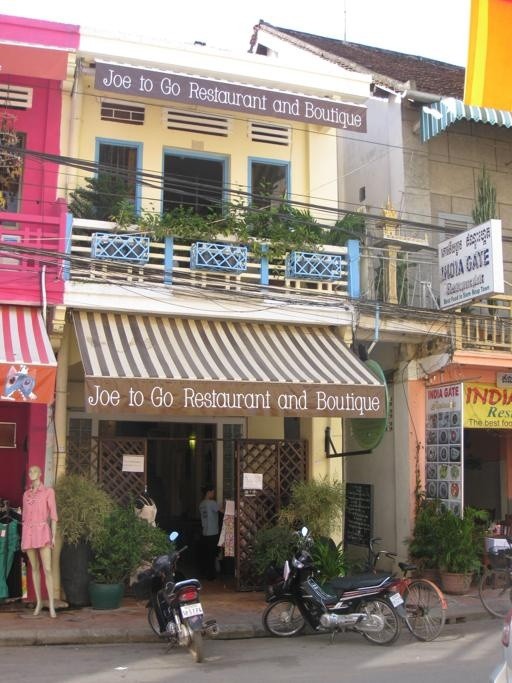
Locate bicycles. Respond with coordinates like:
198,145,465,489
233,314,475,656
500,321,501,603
360,532,452,644
477,522,511,620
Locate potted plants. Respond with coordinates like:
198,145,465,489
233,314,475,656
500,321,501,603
80,506,178,609
247,518,301,580
405,494,442,588
101,213,333,275
435,508,481,593
54,471,107,605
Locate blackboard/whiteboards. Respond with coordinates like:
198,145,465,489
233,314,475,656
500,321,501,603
344,482,374,548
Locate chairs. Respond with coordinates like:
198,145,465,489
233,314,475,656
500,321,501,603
469,504,512,590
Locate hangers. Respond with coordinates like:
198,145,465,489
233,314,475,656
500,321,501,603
0,507,23,524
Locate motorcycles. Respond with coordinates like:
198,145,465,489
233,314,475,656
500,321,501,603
133,531,221,667
261,528,405,646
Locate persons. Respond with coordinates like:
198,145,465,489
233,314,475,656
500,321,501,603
20,465,58,618
129,491,157,587
197,484,225,580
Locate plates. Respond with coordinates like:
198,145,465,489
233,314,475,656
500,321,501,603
427,412,459,514
